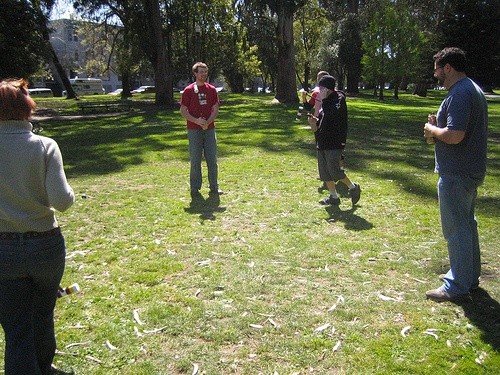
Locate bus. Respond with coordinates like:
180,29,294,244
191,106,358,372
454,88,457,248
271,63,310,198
61,77,106,97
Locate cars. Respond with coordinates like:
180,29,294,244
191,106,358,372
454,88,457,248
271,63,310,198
130,85,155,94
107,88,123,95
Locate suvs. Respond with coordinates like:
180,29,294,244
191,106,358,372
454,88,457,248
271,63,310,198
28,88,53,98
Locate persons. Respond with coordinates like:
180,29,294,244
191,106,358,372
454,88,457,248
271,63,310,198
302,71,345,170
424,47,488,301
0,80,75,375
180,63,223,195
308,76,360,204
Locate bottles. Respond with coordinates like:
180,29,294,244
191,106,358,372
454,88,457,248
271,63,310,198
58,283,80,298
427,111,436,145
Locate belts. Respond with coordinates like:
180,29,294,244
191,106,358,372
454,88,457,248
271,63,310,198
0,226,62,241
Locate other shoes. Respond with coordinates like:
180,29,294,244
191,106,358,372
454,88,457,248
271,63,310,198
191,189,201,196
48,364,74,375
347,183,360,204
426,283,466,302
318,194,340,204
211,189,223,194
439,274,479,289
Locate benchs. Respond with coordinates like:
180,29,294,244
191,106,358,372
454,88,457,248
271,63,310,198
77,100,133,113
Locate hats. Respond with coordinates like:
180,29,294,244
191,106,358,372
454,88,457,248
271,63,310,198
319,75,336,88
